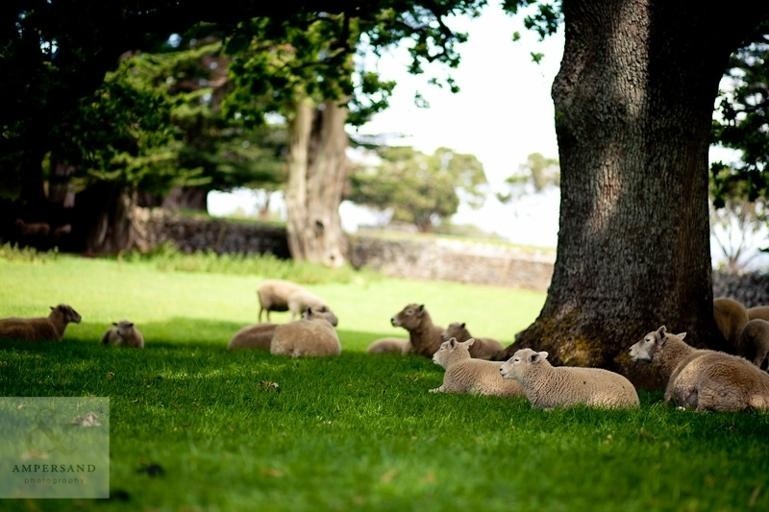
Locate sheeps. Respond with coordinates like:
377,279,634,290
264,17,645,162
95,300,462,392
498,347,641,412
713,297,769,368
427,336,526,398
0,304,82,341
627,325,769,414
225,280,342,359
100,319,145,349
367,301,504,361
